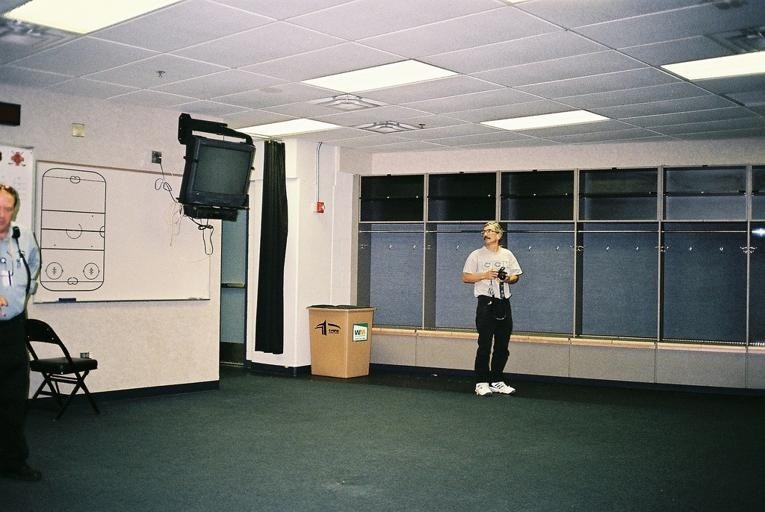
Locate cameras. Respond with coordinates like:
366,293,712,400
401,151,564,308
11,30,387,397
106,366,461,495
496,267,507,280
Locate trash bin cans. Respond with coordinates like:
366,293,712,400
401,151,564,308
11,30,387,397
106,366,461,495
305,305,377,379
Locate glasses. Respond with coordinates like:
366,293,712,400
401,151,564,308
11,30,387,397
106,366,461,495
0,184,16,195
481,229,498,235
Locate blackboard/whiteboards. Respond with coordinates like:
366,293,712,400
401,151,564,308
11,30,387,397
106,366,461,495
0,144,211,304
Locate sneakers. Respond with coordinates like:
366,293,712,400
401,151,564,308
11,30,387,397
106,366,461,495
475,383,492,395
490,382,516,394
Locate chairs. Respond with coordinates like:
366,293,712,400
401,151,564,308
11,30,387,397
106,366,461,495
24,316,102,422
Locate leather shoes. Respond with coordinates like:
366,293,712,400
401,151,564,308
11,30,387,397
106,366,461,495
1,463,41,481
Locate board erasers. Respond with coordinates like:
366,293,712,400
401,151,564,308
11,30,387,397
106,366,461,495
58,298,76,303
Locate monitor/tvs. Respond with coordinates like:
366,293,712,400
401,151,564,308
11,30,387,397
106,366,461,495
183,135,256,208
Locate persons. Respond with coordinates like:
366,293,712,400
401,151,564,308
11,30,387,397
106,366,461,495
1,183,42,482
462,220,524,397
